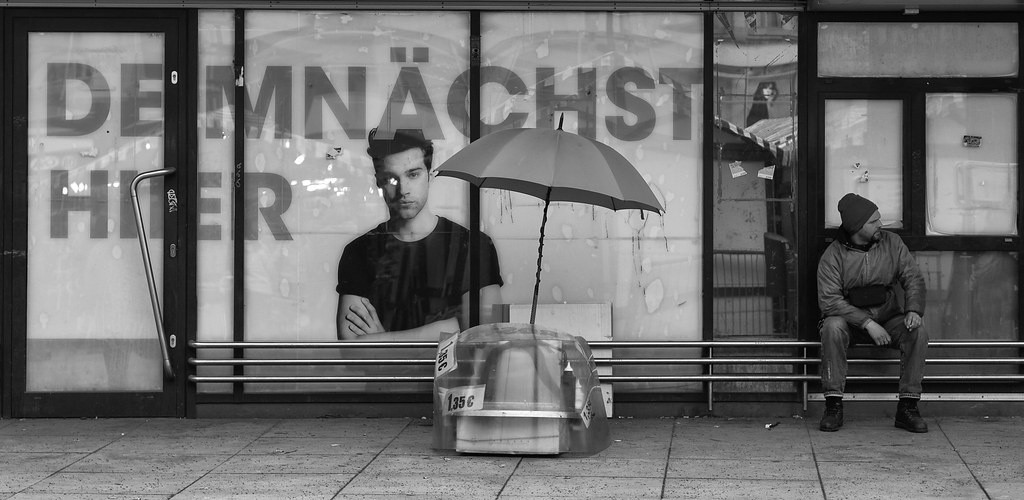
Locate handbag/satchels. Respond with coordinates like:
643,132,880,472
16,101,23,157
848,284,890,308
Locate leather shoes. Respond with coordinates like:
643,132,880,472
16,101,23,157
819,396,844,431
895,399,929,433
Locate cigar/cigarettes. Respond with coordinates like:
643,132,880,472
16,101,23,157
910,325,913,328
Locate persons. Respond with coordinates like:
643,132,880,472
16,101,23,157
817,193,929,433
335,127,504,341
747,81,778,127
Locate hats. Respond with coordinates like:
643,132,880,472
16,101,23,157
837,193,879,236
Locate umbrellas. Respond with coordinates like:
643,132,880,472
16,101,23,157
433,112,666,324
